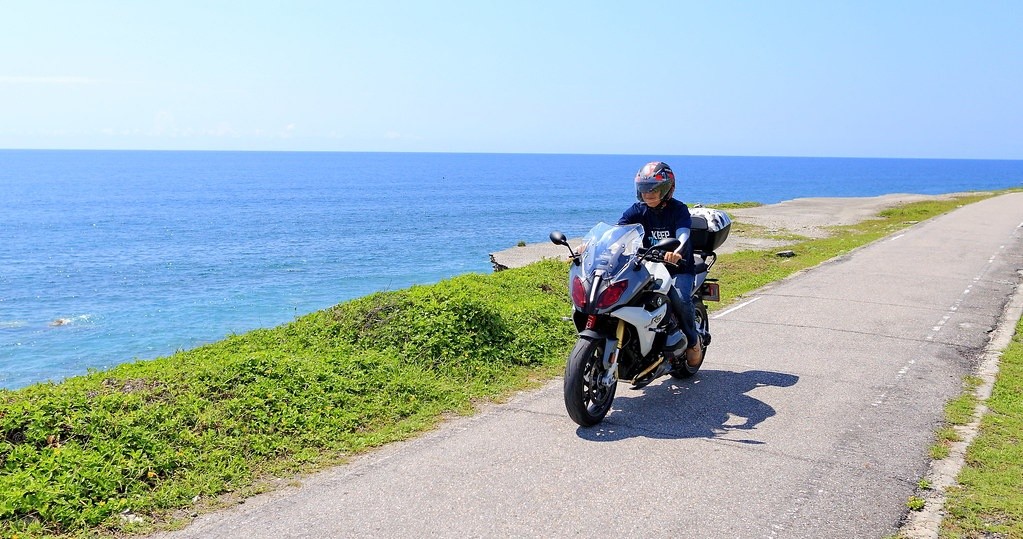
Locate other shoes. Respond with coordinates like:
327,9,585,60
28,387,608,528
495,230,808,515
686,335,703,368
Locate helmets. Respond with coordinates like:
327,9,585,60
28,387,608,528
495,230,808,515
634,161,676,208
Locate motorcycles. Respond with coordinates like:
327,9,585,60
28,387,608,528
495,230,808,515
549,206,731,427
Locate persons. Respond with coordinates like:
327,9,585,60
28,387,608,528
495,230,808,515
577,161,704,367
